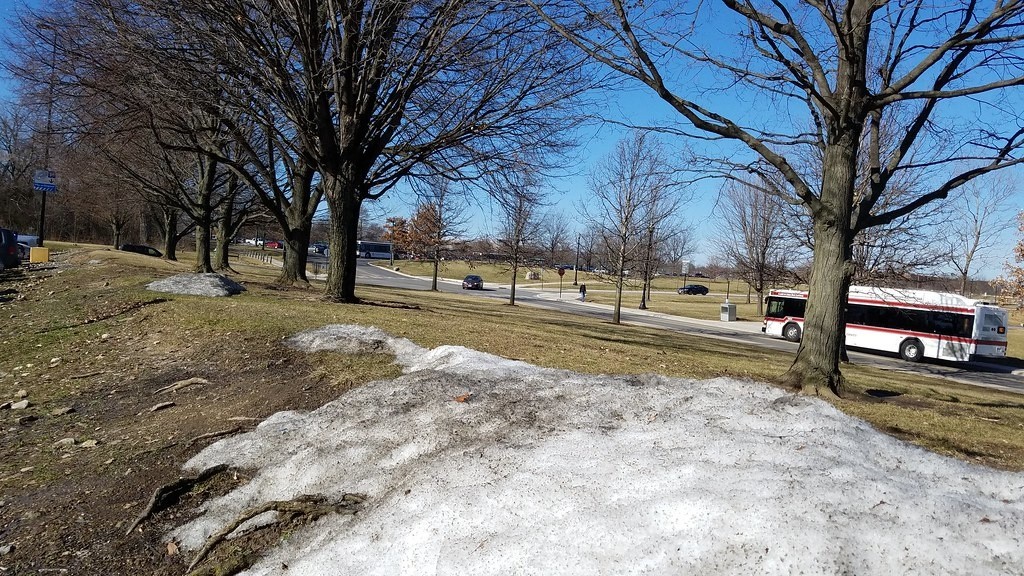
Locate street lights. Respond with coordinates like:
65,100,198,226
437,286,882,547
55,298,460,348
36,20,58,245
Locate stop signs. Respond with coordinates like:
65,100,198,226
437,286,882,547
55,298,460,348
558,268,565,276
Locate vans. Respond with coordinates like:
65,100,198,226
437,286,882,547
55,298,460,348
121,244,162,257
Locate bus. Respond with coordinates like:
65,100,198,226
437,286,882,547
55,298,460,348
357,241,401,259
761,286,1008,365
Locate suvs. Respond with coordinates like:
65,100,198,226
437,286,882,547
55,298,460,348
399,251,411,259
0,227,22,272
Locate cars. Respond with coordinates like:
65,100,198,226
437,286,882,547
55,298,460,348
471,252,712,279
678,285,709,296
461,275,484,290
264,241,282,249
308,244,324,253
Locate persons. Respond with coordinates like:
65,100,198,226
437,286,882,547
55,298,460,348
781,306,784,312
580,283,586,302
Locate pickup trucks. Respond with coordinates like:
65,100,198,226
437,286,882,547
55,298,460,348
17,234,39,248
246,238,264,246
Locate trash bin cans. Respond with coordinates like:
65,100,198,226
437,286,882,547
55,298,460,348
721,304,736,321
